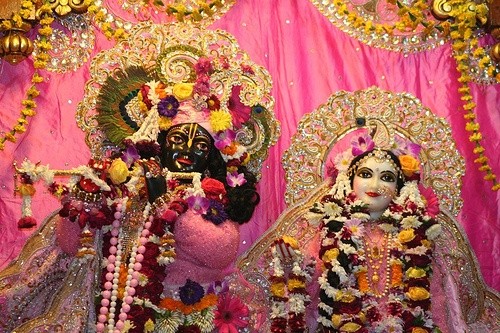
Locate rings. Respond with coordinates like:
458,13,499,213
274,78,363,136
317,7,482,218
145,165,171,179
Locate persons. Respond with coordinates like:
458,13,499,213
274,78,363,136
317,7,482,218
232,87,500,333
0,21,281,333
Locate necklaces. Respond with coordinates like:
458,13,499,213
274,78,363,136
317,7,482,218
96,194,159,333
361,221,394,299
